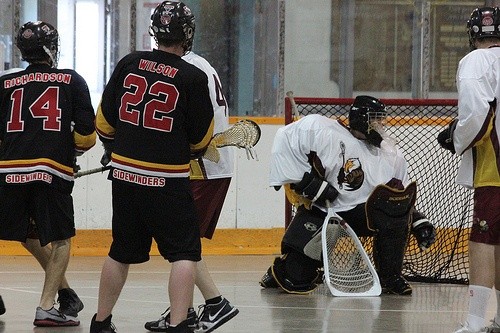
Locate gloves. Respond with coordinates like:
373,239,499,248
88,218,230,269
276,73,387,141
290,172,339,207
411,211,436,251
437,119,459,154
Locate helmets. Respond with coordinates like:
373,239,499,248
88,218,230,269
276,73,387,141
467,6,500,38
349,96,386,130
17,22,59,61
148,0,196,40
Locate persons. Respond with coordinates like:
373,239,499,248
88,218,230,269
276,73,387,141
437,5,500,333
260,95,436,294
0,0,241,333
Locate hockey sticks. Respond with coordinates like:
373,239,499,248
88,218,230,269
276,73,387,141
73,118,263,179
286,90,382,297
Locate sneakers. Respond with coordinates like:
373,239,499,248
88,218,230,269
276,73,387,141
33,306,80,326
90,313,117,333
144,307,198,333
57,288,84,315
193,296,240,333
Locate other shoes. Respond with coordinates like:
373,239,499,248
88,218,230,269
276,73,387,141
382,275,412,295
259,271,277,287
454,318,500,333
